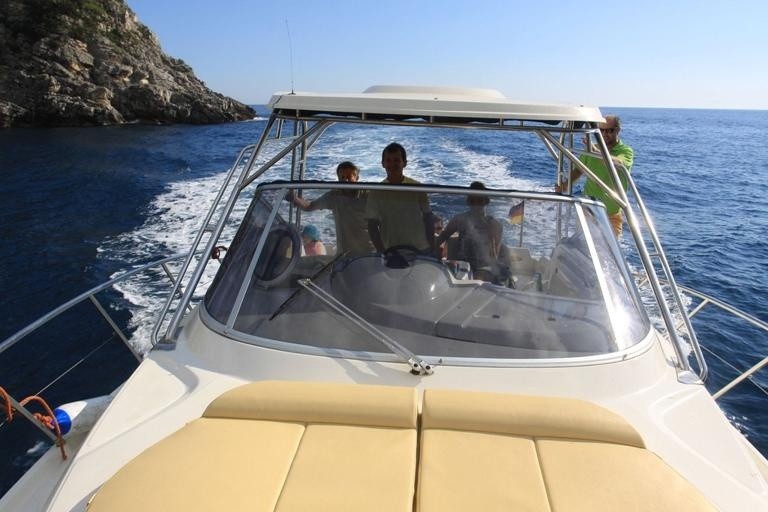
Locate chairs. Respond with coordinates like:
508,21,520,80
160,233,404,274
447,237,517,289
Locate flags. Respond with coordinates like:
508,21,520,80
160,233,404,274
508,200,524,225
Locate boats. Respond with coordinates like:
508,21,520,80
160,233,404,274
0,83,764,509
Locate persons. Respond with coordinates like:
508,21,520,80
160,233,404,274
300,225,327,257
361,142,435,258
430,181,504,283
430,214,450,260
284,161,378,255
553,114,635,241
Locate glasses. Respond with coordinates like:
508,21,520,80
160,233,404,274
600,127,619,134
